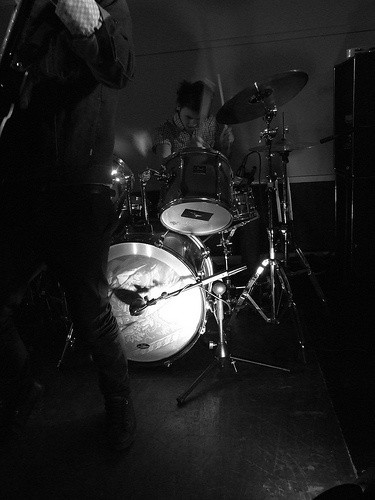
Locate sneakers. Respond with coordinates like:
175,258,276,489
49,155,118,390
104,392,139,452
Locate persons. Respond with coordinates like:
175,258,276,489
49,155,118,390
152,80,245,282
0,0,138,450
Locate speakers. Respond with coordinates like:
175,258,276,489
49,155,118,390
334,51,375,287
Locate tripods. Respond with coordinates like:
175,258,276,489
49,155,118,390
176,121,329,404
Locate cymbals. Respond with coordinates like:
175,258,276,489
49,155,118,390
249,141,319,154
216,69,309,125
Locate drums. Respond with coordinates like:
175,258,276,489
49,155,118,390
99,153,138,228
106,228,214,366
230,175,261,227
155,148,236,234
